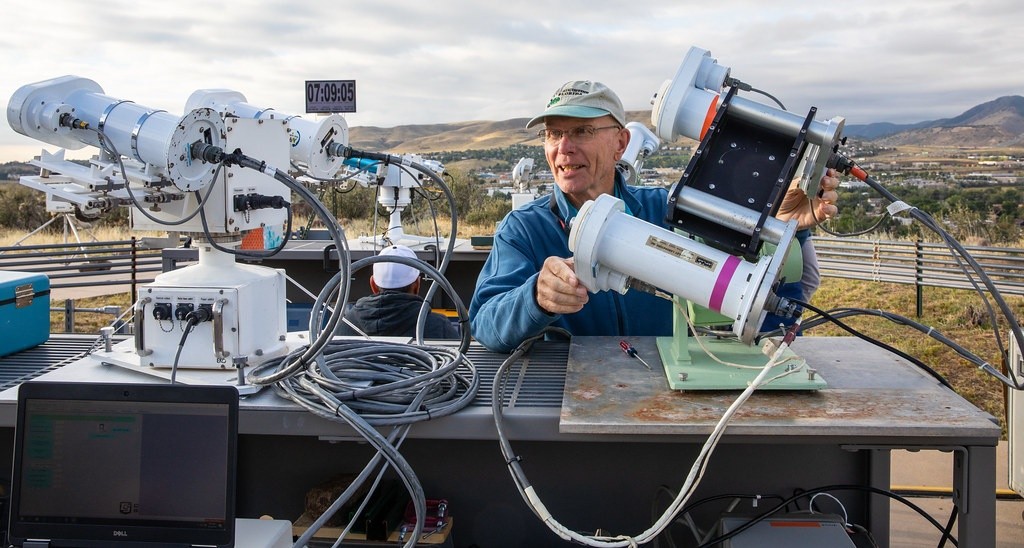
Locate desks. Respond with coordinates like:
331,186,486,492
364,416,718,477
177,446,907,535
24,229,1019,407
0,238,1002,548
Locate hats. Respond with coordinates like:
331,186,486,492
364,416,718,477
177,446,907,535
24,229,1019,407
526,80,625,129
373,244,421,289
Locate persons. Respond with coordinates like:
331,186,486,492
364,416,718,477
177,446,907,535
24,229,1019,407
335,245,460,339
468,80,839,353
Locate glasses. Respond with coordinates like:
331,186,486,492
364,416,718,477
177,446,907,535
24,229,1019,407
537,125,622,146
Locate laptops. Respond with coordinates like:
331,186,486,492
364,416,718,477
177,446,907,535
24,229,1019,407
8,381,239,548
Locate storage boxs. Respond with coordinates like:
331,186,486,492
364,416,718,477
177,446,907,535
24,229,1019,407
1,273,51,358
